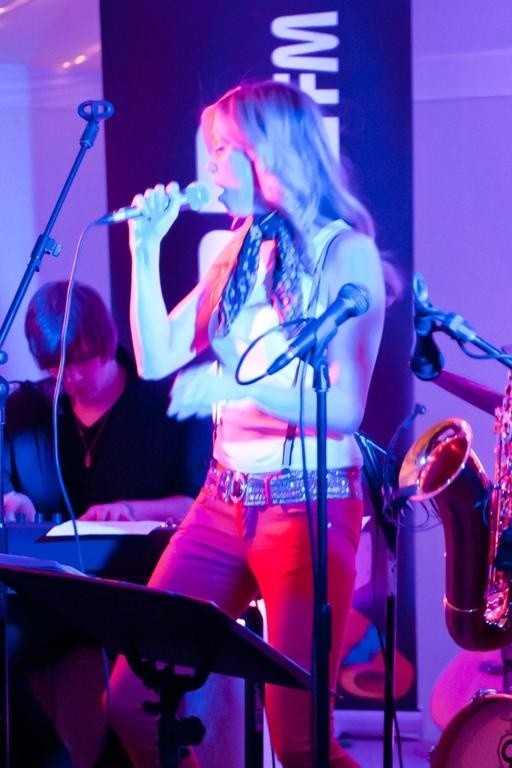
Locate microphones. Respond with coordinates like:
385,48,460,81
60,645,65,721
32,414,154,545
112,180,211,223
411,286,445,381
269,281,371,374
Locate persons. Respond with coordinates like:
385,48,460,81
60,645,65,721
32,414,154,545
0,281,214,768
100,80,384,768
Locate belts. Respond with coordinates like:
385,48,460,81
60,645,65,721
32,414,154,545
201,460,366,512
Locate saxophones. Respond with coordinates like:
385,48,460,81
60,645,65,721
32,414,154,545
397,419,512,651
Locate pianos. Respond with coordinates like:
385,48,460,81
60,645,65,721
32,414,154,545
0,514,178,587
